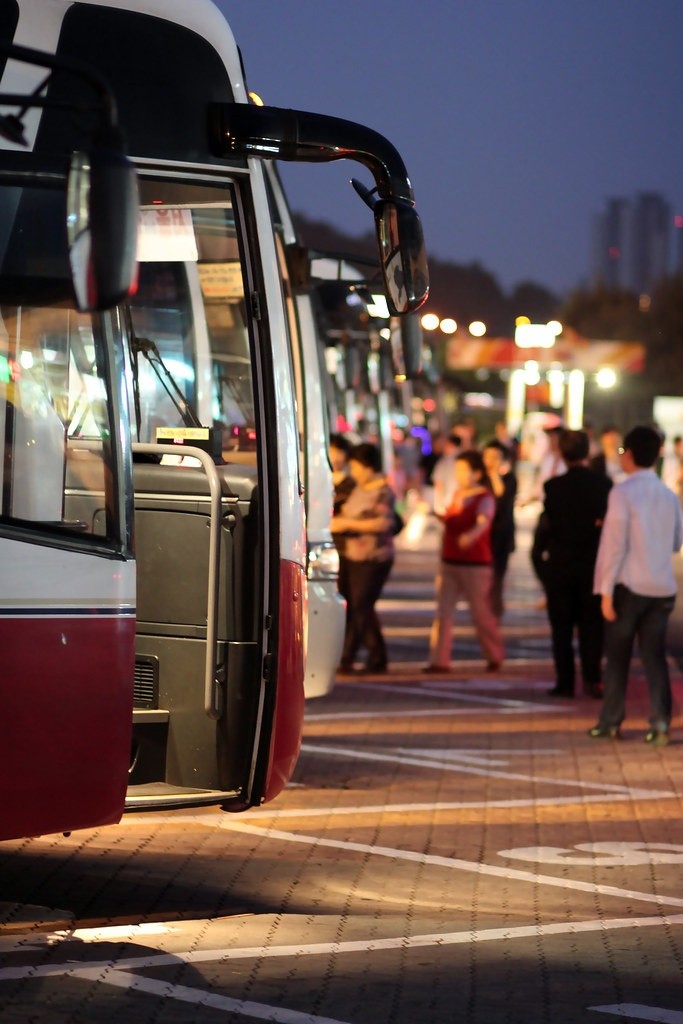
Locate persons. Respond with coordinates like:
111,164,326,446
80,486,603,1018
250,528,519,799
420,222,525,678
329,441,397,674
528,431,616,699
328,434,356,596
587,426,683,746
482,439,516,626
351,414,683,534
419,450,506,675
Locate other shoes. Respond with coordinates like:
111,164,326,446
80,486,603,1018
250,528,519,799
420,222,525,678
482,662,507,674
420,665,455,673
584,678,606,698
587,723,620,740
546,685,575,697
644,729,670,746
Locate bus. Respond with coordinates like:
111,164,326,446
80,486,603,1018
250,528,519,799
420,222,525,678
0,0,433,850
